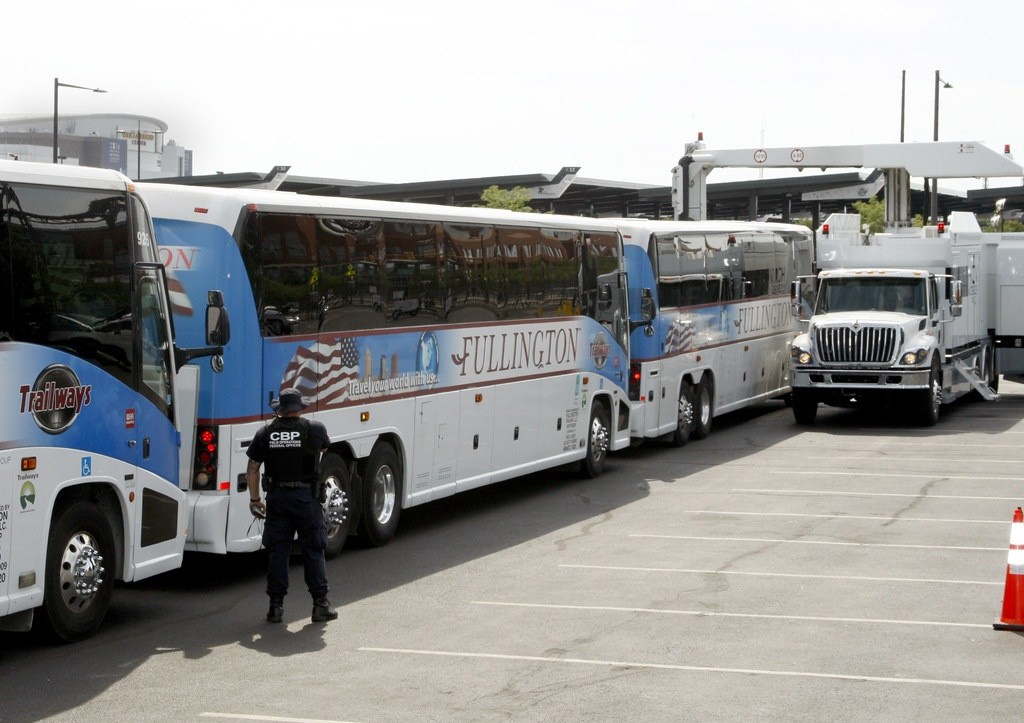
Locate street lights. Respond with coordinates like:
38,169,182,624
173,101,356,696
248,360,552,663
116,120,164,182
53,77,108,164
932,70,953,225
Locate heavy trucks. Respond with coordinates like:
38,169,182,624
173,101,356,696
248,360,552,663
789,212,1024,428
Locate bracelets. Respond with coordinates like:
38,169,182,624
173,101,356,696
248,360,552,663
250,497,261,502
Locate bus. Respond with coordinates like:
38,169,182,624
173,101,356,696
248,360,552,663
597,218,817,448
0,158,231,647
135,182,656,560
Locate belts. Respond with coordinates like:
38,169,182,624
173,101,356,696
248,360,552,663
277,481,311,488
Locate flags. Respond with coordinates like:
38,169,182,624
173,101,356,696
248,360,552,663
278,337,362,412
664,316,697,354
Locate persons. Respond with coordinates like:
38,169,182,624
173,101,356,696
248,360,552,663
245,386,339,624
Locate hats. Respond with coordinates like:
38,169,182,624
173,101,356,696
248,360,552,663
270,388,312,413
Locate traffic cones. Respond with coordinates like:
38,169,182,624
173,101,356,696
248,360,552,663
991,506,1024,632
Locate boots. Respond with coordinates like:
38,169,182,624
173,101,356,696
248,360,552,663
310,590,338,622
267,593,284,624
